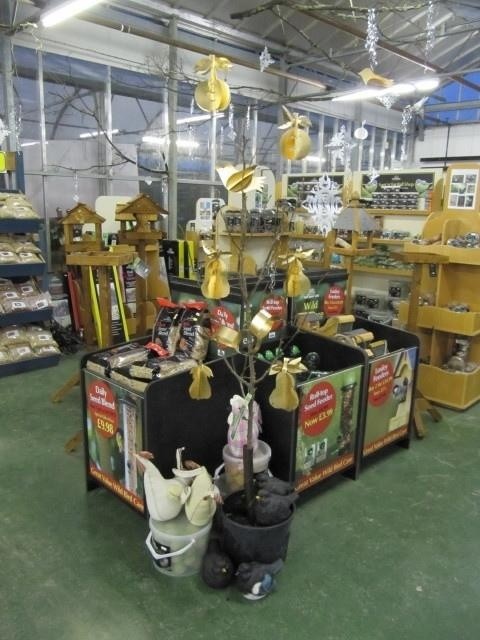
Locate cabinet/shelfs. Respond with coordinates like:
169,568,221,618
294,316,421,480
80,333,240,518
398,164,480,412
1,151,60,377
242,332,367,502
216,178,443,333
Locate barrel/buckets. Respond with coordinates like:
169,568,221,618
223,440,271,492
145,517,212,577
